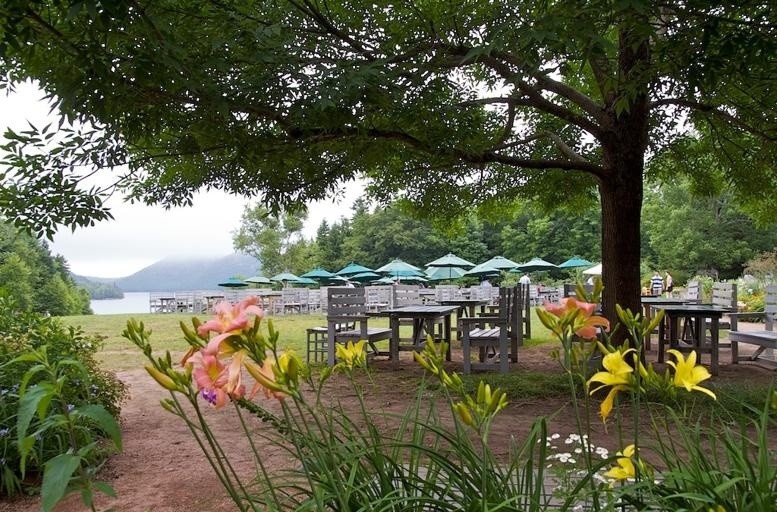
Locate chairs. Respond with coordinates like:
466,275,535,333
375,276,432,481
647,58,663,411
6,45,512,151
471,282,531,362
565,281,775,371
394,286,467,306
306,296,356,362
327,286,392,369
457,286,509,375
150,286,327,316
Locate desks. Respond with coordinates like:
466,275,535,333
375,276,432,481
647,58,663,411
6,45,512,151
381,306,460,370
651,306,729,377
435,300,491,341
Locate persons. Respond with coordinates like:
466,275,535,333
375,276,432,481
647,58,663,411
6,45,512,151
649,271,665,294
665,271,675,299
